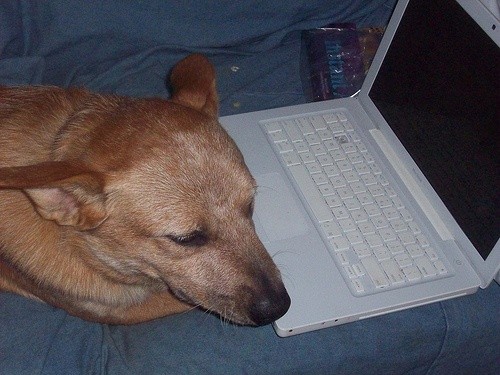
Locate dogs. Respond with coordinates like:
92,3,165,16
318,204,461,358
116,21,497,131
0,51,292,329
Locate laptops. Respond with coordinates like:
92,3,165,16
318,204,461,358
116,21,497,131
217,0,500,336
